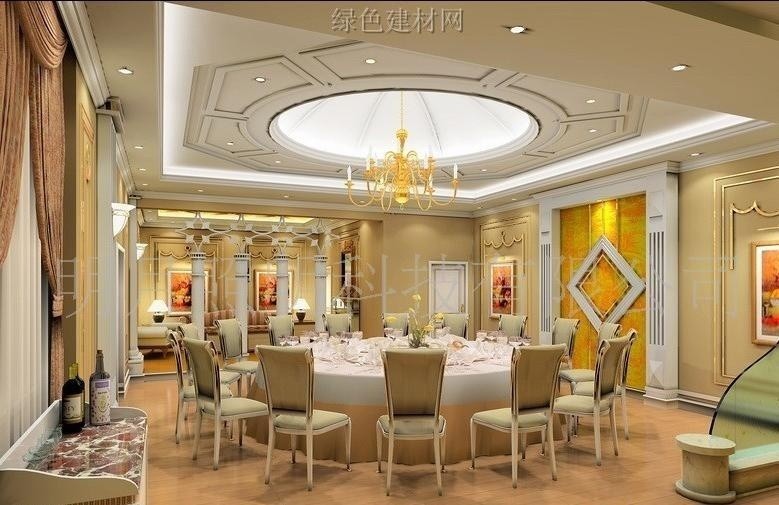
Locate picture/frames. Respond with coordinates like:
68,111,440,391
751,238,779,346
487,260,517,320
166,268,210,317
254,269,295,317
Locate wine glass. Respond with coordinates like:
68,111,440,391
22,423,62,473
277,327,535,371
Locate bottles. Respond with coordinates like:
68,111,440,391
60,362,85,434
89,349,111,426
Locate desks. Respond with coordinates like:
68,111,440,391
1,399,149,505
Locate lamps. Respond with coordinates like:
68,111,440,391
148,300,168,323
137,243,148,260
292,300,311,321
112,203,136,237
345,91,460,212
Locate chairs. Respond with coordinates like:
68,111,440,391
138,322,183,357
